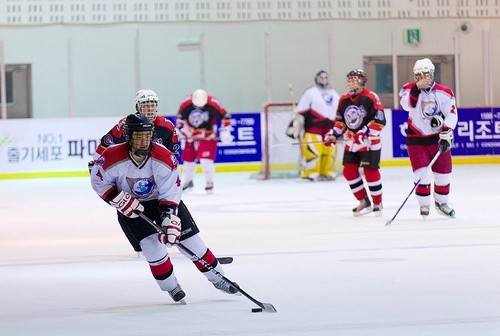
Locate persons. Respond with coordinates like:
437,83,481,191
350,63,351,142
324,68,386,213
176,90,231,190
286,71,341,181
91,114,239,302
94,90,180,252
399,58,458,216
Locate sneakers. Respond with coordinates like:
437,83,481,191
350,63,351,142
420,206,430,220
168,283,187,305
372,203,383,217
435,201,455,218
205,182,213,194
212,278,242,296
352,195,372,216
182,180,193,193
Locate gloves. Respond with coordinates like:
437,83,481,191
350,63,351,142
324,129,342,146
88,161,94,175
110,190,144,219
438,129,454,152
355,125,370,144
157,214,181,248
175,128,186,142
219,128,233,143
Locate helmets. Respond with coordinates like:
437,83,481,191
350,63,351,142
413,58,435,83
192,89,207,107
123,114,154,156
315,71,328,88
346,69,367,93
134,90,159,122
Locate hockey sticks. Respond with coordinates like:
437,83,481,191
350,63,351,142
131,210,276,313
177,136,223,142
288,83,307,161
382,145,442,227
270,137,382,148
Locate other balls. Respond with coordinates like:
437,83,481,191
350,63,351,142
251,308,263,312
